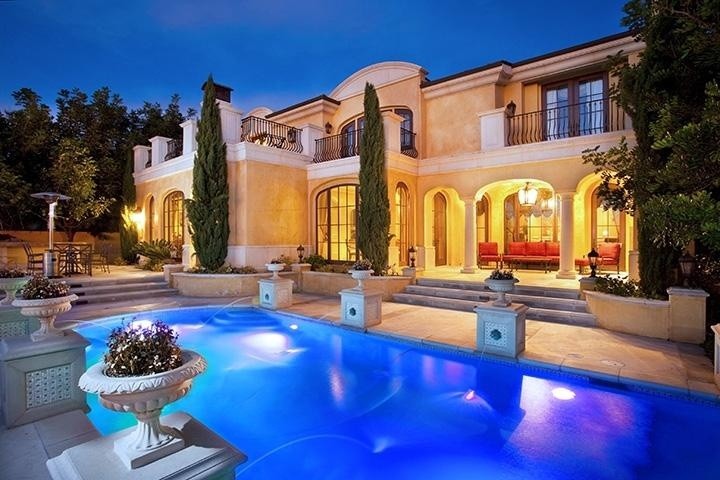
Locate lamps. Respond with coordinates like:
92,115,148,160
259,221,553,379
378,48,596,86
518,181,540,207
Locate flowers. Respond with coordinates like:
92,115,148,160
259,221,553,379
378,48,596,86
16,274,71,299
270,259,282,263
98,318,182,377
351,258,372,270
488,269,514,281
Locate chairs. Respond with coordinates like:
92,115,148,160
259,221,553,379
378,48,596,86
22,241,44,274
87,241,111,275
595,243,623,275
478,242,560,274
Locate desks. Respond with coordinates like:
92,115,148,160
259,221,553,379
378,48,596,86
52,243,93,277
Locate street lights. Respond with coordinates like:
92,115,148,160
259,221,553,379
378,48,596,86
30,191,72,253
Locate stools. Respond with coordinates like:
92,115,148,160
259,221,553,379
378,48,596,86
575,259,589,275
